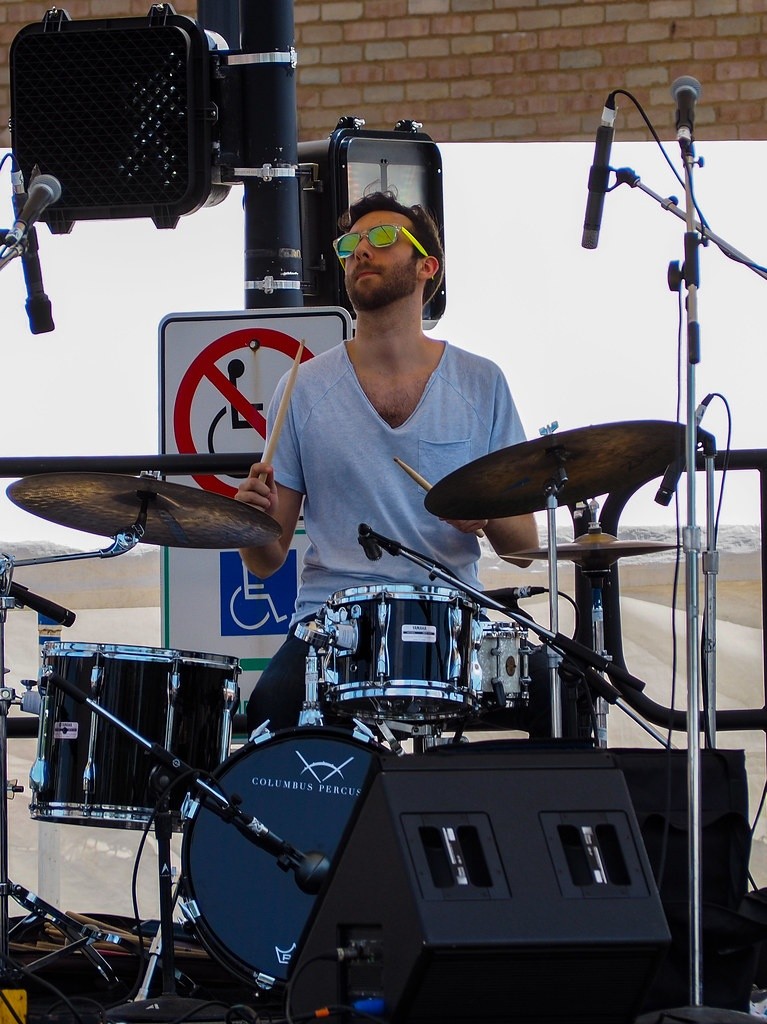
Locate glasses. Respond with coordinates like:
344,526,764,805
333,223,435,281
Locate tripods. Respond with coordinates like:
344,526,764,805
0,537,198,1001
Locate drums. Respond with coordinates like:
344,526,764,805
181,726,400,998
28,639,241,830
476,618,535,718
323,584,480,727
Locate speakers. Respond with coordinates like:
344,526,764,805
288,751,672,1024
616,746,747,907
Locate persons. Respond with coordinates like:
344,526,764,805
234,191,538,740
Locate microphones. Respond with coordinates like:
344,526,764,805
11,162,55,334
8,581,76,629
5,173,62,247
671,76,701,148
581,96,615,249
480,587,545,602
655,393,716,507
359,524,383,561
296,853,331,895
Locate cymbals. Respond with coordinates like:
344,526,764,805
423,417,686,521
500,532,683,566
5,469,280,550
